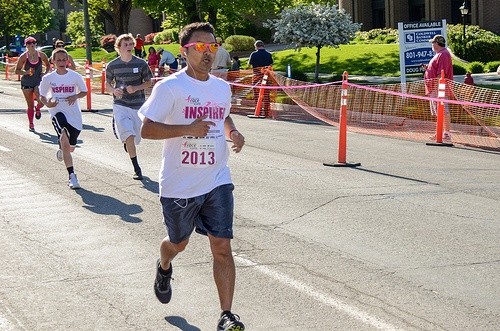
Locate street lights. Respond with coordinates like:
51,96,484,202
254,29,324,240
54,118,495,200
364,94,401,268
459,2,470,59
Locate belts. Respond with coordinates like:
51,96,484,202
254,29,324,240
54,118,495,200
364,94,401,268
213,67,224,70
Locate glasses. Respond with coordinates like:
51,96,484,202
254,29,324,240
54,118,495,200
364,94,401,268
54,59,67,61
183,42,220,52
57,45,64,48
27,42,37,45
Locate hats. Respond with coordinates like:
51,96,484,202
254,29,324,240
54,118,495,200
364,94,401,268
429,35,446,44
466,71,472,74
156,47,162,55
216,37,222,43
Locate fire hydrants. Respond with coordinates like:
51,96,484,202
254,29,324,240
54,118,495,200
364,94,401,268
462,71,476,110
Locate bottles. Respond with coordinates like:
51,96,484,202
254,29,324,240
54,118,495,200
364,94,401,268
155,64,158,77
29,66,35,76
49,63,54,71
115,81,125,100
48,97,59,103
68,62,72,69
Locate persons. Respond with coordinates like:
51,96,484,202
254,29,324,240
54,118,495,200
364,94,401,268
248,40,274,117
15,37,51,132
157,47,178,74
134,34,145,58
56,40,76,70
211,37,231,71
40,49,88,188
106,33,154,180
136,22,245,331
422,35,454,142
147,47,161,74
230,56,240,78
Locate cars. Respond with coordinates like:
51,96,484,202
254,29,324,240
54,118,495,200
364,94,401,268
0,45,25,57
40,45,54,56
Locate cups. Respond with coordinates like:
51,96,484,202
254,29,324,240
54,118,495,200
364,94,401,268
230,60,233,65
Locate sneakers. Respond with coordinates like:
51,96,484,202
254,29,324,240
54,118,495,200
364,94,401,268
124,143,127,152
133,167,142,179
29,123,34,131
56,149,64,162
35,106,41,119
430,132,451,140
67,173,79,188
154,258,173,304
217,311,245,331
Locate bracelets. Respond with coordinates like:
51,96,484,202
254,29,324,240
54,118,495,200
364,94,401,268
230,130,237,139
